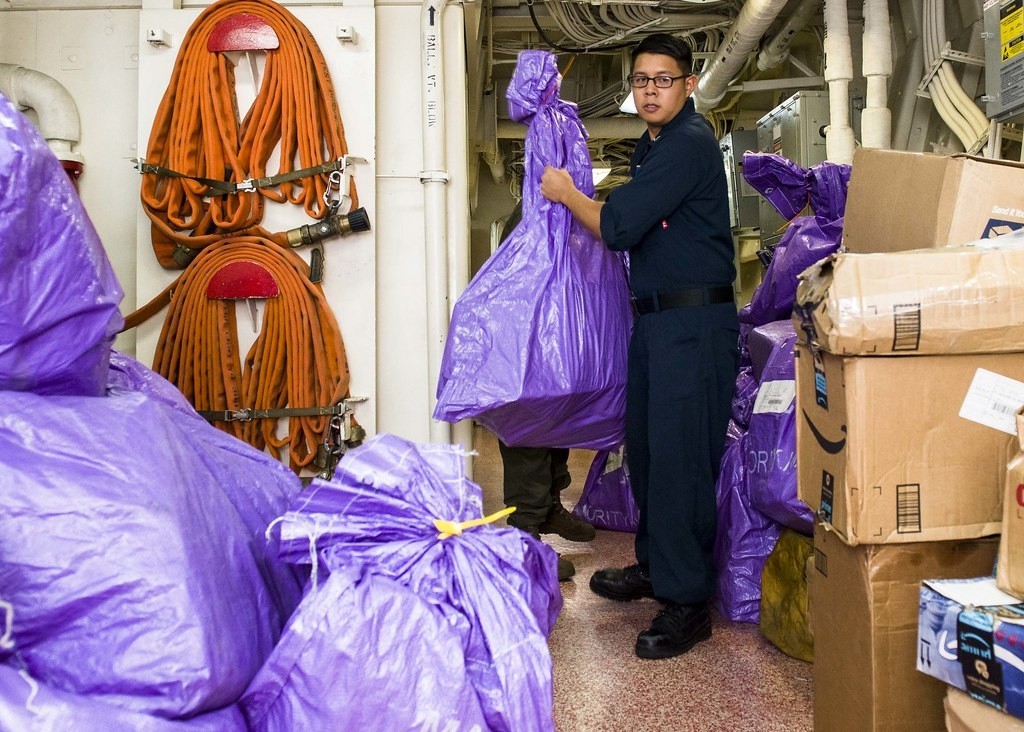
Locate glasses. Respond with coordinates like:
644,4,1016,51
627,73,693,88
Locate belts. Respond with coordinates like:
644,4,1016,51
633,286,734,314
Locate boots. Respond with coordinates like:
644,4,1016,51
537,491,596,542
521,526,576,580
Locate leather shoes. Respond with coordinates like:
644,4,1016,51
589,564,664,603
635,604,714,659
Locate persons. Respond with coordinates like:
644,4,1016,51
539,30,742,661
497,174,596,581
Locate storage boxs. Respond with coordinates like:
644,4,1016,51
759,148,1024,732
